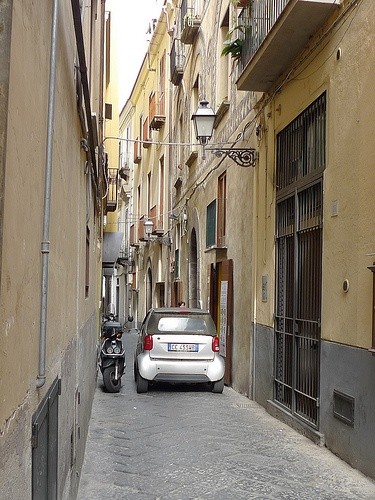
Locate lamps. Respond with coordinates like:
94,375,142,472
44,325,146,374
191,98,219,146
144,218,154,238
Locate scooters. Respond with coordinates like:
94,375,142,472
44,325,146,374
100,312,134,393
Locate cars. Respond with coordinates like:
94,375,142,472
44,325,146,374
134,307,225,395
